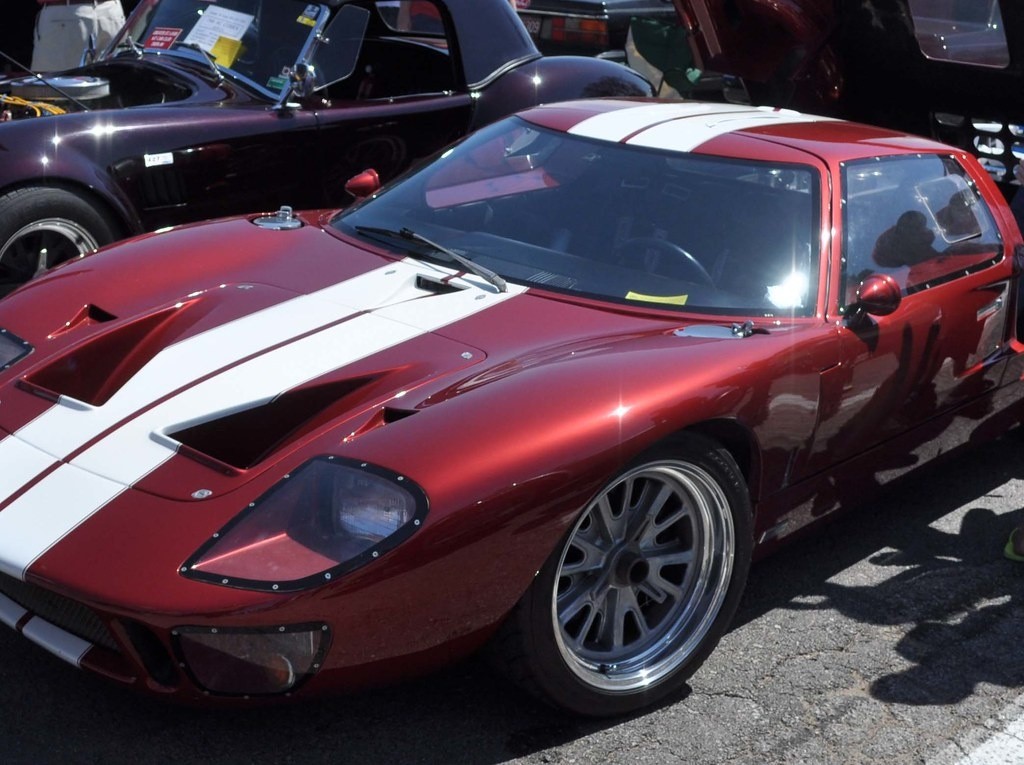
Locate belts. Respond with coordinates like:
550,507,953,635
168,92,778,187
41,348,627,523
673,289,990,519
45,0,108,7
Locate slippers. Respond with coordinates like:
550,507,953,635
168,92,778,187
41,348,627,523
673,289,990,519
1004,526,1024,561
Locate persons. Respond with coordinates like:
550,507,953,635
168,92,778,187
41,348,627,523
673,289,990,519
936,189,982,235
874,211,938,265
397,0,444,34
27,0,140,71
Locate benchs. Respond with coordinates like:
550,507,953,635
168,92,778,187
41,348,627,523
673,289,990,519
272,37,453,100
612,161,880,325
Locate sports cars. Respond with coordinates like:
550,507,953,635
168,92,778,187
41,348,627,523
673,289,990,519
1,99,1024,712
0,1,655,291
